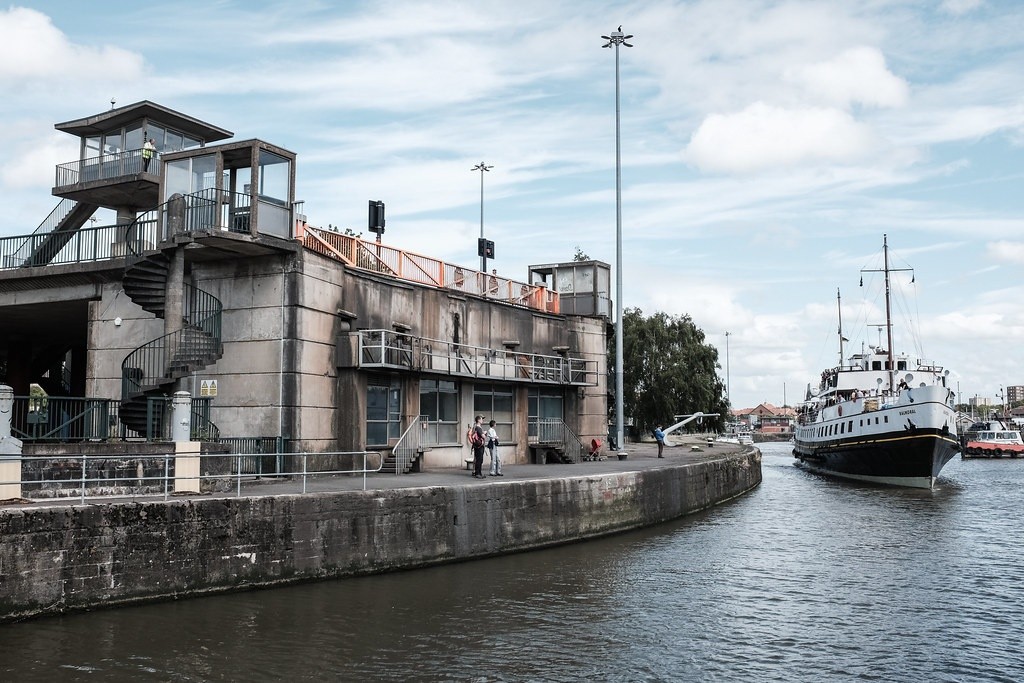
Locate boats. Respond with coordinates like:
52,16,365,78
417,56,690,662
737,430,755,447
961,426,1024,457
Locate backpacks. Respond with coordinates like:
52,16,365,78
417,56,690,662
467,428,473,444
471,426,484,450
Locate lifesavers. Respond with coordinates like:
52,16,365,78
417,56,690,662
966,446,1003,457
1011,450,1018,458
838,405,843,415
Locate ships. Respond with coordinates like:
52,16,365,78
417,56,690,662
791,233,963,491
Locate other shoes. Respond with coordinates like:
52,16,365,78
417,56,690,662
658,456,665,458
475,474,483,478
490,473,497,476
497,472,504,476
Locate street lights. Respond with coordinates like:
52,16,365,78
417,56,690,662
724,332,733,406
596,23,637,456
472,161,493,271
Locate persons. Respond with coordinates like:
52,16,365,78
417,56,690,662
655,425,665,458
487,420,503,476
897,379,909,391
142,139,156,172
471,413,488,478
797,389,864,414
